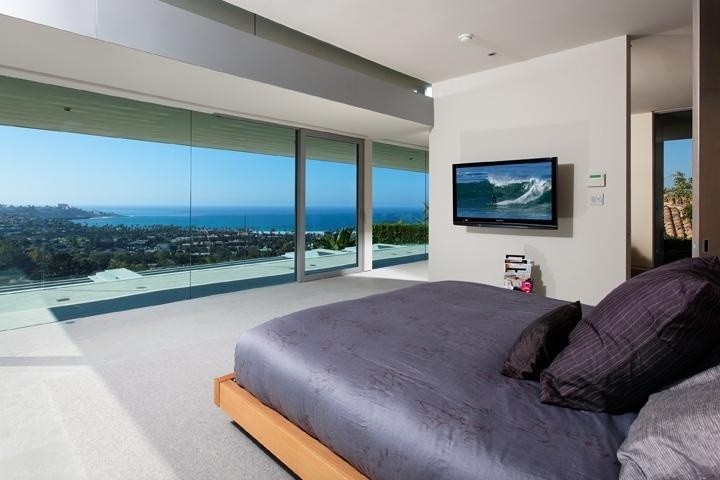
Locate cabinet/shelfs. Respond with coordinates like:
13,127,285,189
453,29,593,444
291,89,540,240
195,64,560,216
504,253,537,296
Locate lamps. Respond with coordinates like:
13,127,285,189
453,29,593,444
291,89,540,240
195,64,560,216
458,33,473,42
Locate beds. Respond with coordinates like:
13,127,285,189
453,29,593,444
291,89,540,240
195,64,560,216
214,281,720,480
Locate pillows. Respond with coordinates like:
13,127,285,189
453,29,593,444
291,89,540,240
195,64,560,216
539,256,719,412
616,367,720,479
503,300,584,380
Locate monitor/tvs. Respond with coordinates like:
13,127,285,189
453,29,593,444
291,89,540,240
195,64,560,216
452,157,557,230
